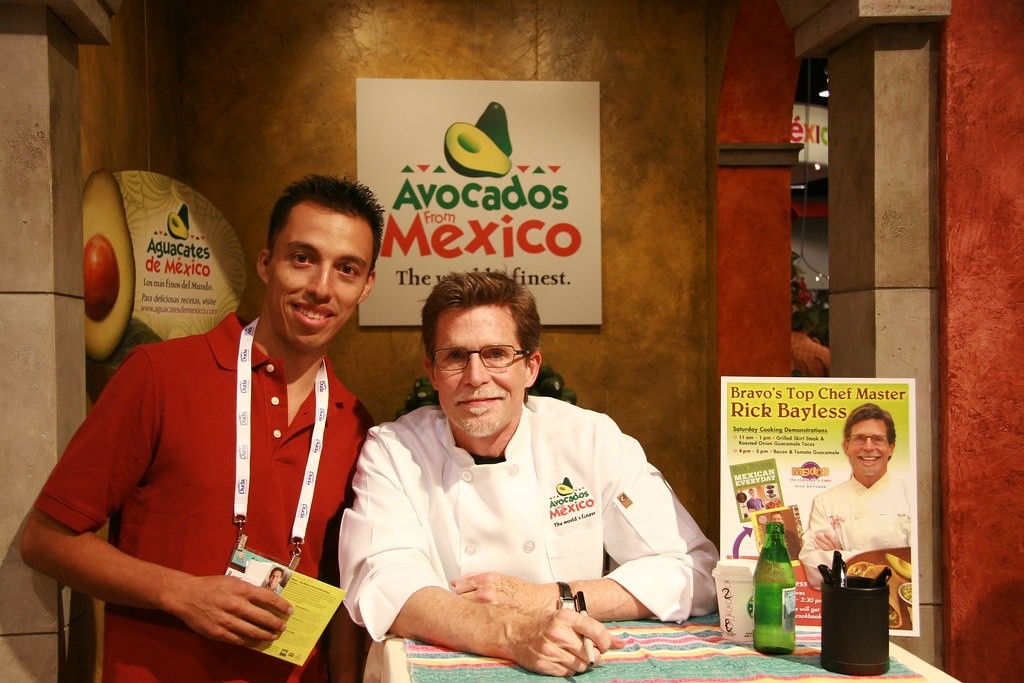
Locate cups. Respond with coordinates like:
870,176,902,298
820,577,890,676
712,557,757,643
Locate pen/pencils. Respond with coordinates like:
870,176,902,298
573,590,596,665
817,551,892,589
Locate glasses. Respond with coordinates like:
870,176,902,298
848,434,888,446
431,345,529,372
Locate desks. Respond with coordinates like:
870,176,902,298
364,608,963,683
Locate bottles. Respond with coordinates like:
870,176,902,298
752,523,796,655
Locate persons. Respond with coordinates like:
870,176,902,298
771,511,800,561
790,284,831,377
263,567,284,594
746,488,765,512
798,402,911,591
337,272,719,683
19,173,384,682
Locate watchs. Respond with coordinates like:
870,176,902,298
557,581,574,611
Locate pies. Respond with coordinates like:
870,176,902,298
846,561,902,629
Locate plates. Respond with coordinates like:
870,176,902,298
898,582,913,604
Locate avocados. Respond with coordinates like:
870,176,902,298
886,553,911,579
79,169,136,363
